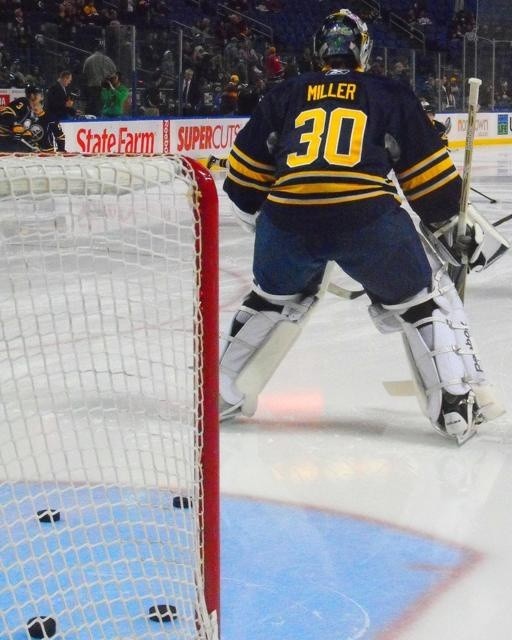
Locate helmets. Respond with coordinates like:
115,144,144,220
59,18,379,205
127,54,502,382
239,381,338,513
25,82,41,94
313,9,372,71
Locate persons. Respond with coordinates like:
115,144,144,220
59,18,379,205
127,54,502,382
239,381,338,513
0,0,512,152
218,9,510,445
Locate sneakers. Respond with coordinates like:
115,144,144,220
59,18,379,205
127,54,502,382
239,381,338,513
440,395,478,436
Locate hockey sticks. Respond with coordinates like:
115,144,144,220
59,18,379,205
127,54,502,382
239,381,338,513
326,214,512,300
382,78,483,398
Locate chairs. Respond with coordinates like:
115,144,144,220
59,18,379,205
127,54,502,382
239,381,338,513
1,1,510,160
